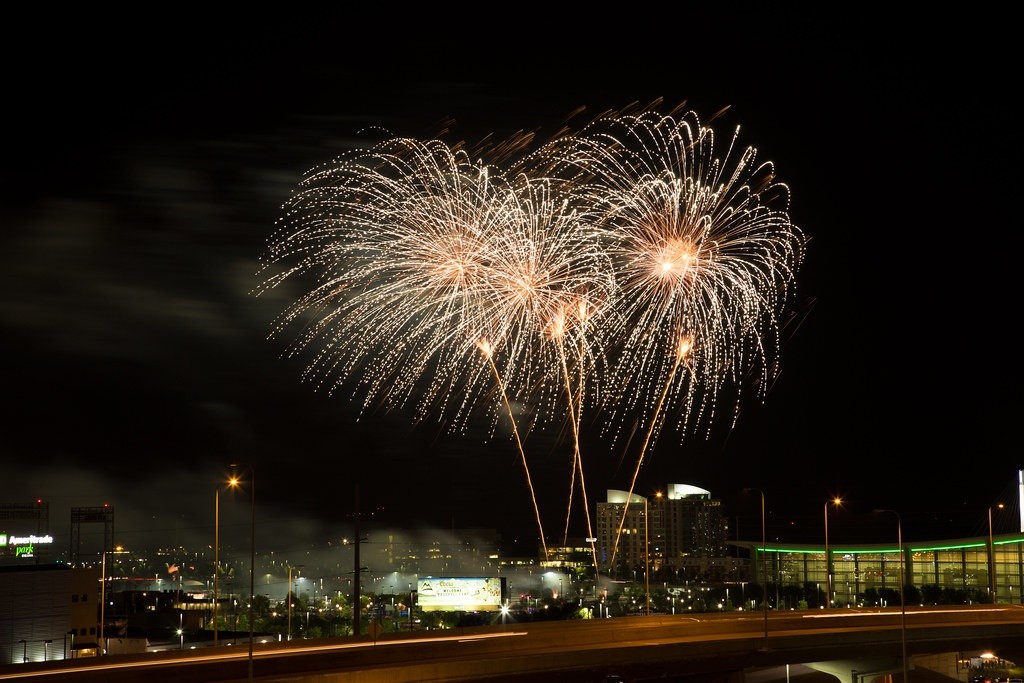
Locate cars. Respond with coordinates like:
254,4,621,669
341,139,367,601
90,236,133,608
968,673,1000,683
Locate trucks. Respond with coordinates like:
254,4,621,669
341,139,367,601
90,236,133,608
641,607,671,615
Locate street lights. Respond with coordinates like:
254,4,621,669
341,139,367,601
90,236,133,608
409,583,412,631
645,493,661,615
875,508,909,683
100,547,121,657
825,499,840,608
744,487,768,638
230,468,254,683
289,567,295,634
214,479,238,642
